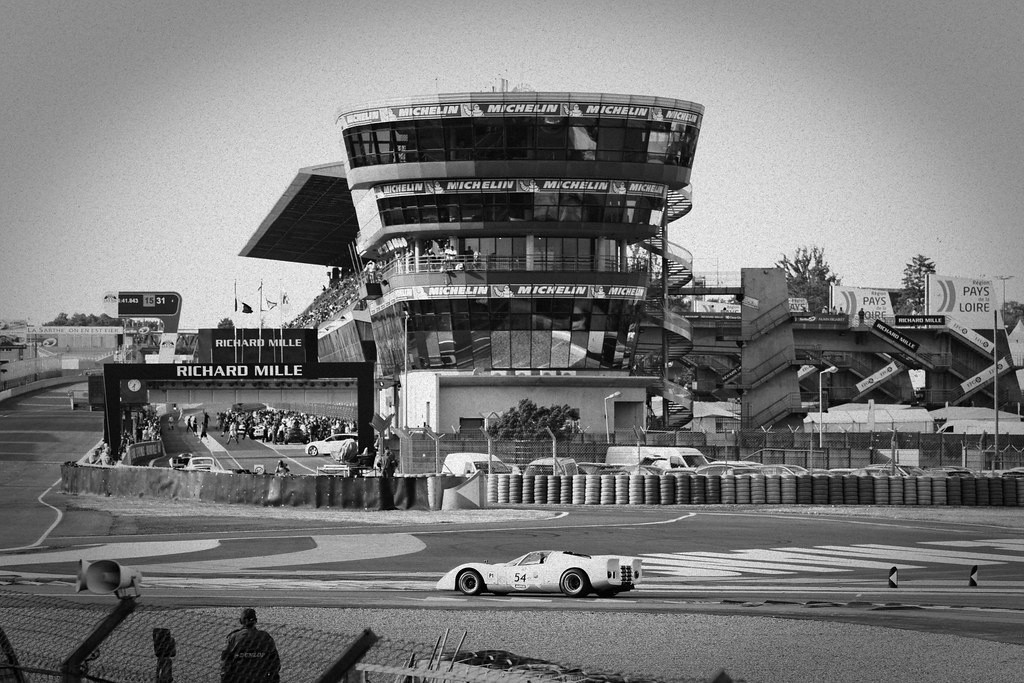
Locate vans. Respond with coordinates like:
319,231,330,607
521,457,579,477
441,451,512,476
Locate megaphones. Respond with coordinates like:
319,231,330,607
86,560,142,595
75,558,93,593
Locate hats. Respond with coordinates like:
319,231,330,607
239,609,256,624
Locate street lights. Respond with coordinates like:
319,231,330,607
818,366,840,448
603,390,624,444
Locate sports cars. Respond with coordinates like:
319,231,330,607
436,549,643,598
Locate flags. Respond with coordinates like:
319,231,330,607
267,300,277,310
240,302,254,313
282,293,290,304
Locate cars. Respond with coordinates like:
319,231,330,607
575,460,985,477
186,456,217,472
305,433,359,457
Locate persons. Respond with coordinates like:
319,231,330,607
710,305,716,313
858,307,865,322
177,408,185,421
220,608,281,683
821,306,828,314
193,418,199,436
422,244,481,270
377,446,398,477
185,416,193,432
88,409,160,465
203,409,211,428
839,307,844,313
167,414,174,430
215,412,222,430
200,422,208,441
220,407,357,445
282,247,415,327
831,306,837,315
911,308,917,315
274,459,291,476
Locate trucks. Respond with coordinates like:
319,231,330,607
604,445,711,470
